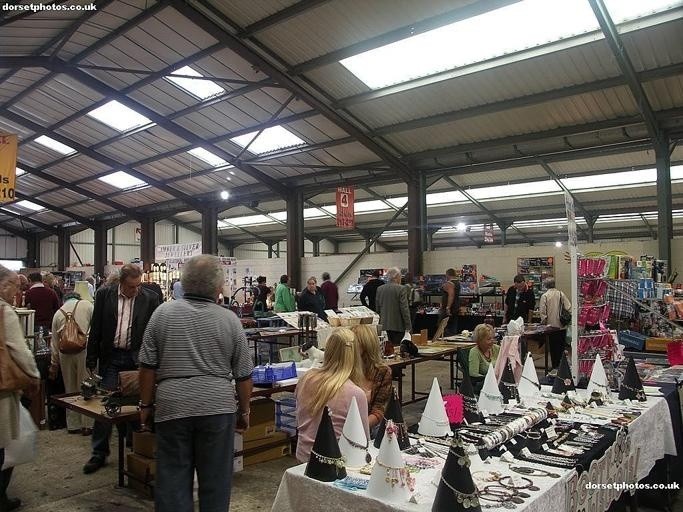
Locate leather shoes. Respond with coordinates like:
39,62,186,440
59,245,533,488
1,494,21,512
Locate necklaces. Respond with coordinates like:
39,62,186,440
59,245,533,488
311,376,647,511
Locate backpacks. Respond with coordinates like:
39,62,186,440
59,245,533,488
58,299,89,354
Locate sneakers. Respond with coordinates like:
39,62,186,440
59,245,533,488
83,457,106,474
67,428,90,436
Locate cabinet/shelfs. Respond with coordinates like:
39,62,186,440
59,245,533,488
139,260,194,302
346,255,557,323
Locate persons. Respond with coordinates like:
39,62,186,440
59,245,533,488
0,264,41,512
0,262,573,476
137,254,253,512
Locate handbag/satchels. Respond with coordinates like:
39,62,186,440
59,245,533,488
0,305,40,393
558,291,571,327
1,402,38,470
